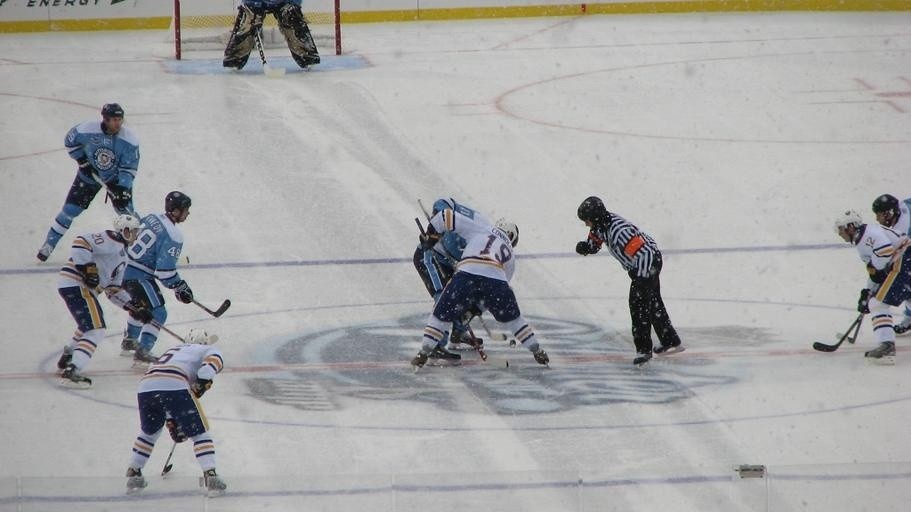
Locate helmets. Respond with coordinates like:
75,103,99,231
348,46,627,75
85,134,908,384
872,194,898,212
496,217,518,244
101,103,124,118
114,214,140,243
185,328,208,344
165,191,191,212
577,196,605,225
835,209,862,228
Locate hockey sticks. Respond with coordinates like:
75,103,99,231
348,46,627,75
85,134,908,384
160,443,174,477
836,310,868,344
417,197,509,342
240,1,285,77
155,272,230,317
415,217,508,367
812,302,868,352
96,283,219,346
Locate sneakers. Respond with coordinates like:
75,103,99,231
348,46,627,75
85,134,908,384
57,340,158,384
36,243,54,260
411,329,483,368
653,335,681,353
894,322,911,333
126,469,146,488
205,476,227,491
534,349,549,364
864,341,895,358
634,352,652,364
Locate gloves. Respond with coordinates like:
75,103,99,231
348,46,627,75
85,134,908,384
82,262,99,287
166,418,187,442
175,280,193,303
132,306,152,324
191,375,212,398
77,155,99,181
576,241,590,256
858,289,870,314
111,185,130,208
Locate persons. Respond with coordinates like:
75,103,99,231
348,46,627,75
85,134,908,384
572,194,682,365
220,0,322,70
412,196,520,361
51,212,154,384
831,205,911,359
856,193,911,334
411,207,550,369
107,189,195,364
123,326,226,493
37,102,141,259
